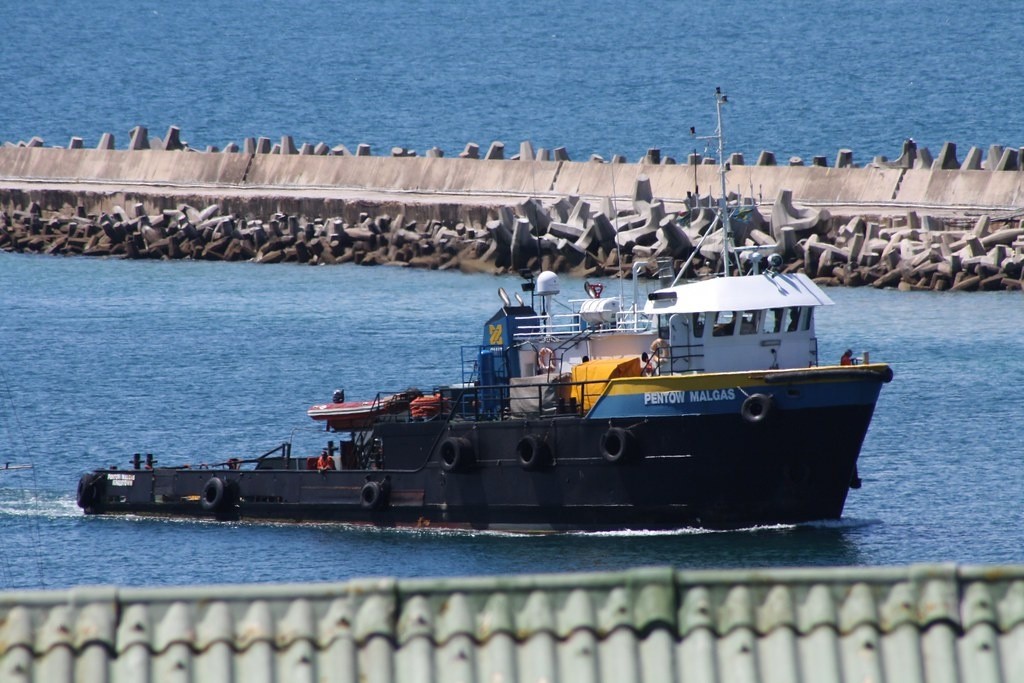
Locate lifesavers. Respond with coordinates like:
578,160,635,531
76,473,95,509
359,481,384,510
438,435,462,471
535,347,558,372
739,393,776,424
600,426,633,465
650,338,670,365
514,433,541,470
199,477,233,511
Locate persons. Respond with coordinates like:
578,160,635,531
317,451,336,473
840,349,853,366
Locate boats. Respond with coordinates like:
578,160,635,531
75,83,893,532
